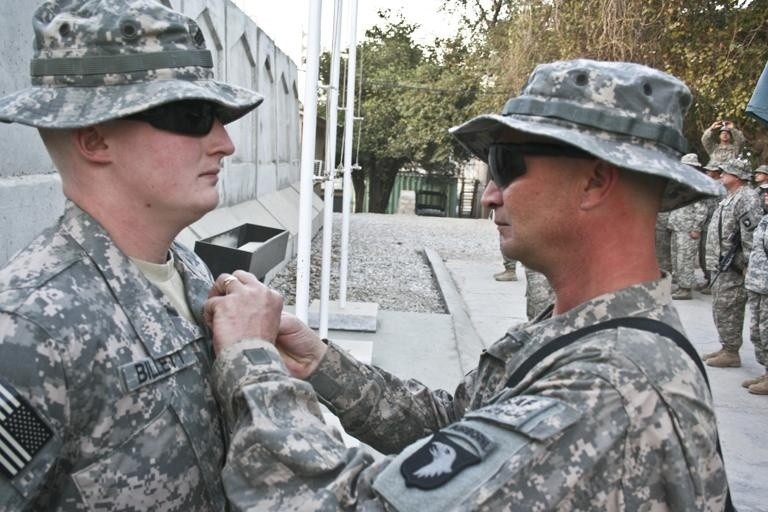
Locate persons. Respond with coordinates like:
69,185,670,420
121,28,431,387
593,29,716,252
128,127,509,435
493,236,555,322
697,120,768,295
741,184,767,395
0,0,263,512
656,153,707,300
700,160,764,368
203,58,739,512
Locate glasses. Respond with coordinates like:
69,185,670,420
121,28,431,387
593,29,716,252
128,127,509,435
487,141,593,188
120,100,217,136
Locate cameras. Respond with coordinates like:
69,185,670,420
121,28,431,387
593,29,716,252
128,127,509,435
721,121,727,125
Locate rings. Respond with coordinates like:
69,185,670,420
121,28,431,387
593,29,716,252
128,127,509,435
222,274,238,293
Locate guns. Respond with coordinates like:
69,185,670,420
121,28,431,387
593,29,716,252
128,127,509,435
708,232,740,289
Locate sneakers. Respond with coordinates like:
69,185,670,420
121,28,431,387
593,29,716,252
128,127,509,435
742,372,768,396
497,269,517,281
703,349,741,367
672,285,691,299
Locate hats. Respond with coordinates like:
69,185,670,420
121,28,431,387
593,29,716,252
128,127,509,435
719,125,731,132
2,0,264,129
681,153,767,190
450,58,726,213
